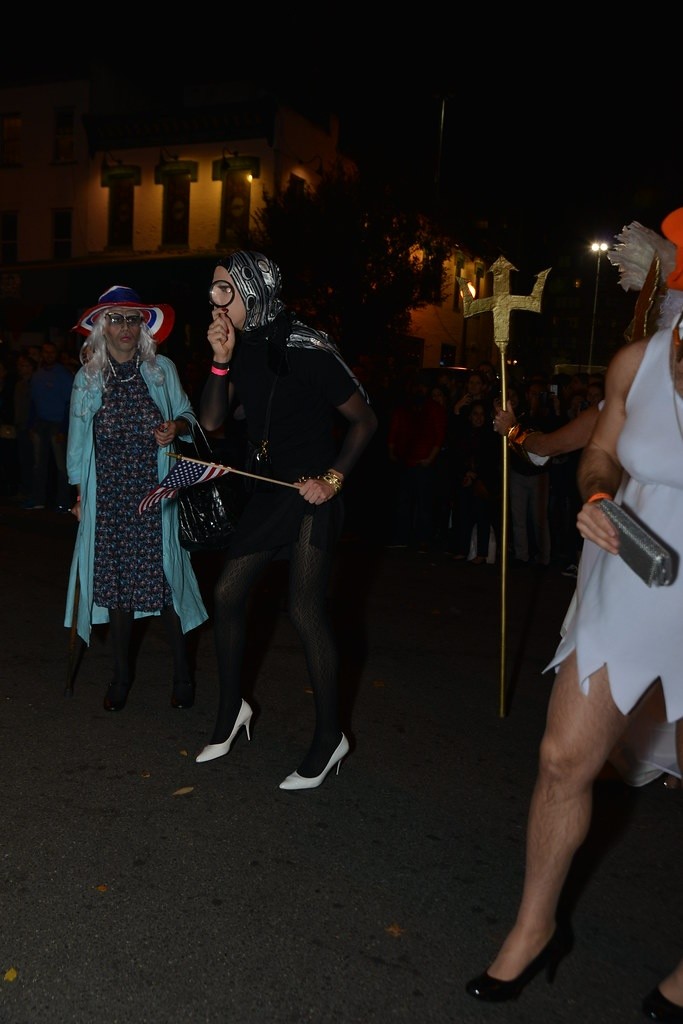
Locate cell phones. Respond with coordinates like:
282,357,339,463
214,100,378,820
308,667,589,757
550,385,558,395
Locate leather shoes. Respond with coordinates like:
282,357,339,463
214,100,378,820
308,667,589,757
101,672,131,713
170,676,197,712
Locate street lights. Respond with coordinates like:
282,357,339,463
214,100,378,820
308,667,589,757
586,239,611,377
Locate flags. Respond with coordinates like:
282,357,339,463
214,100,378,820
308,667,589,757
137,459,236,515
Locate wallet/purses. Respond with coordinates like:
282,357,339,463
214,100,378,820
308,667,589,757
600,498,675,587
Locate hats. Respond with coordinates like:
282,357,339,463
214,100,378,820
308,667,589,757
75,283,175,347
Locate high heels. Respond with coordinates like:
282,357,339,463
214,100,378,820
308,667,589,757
196,698,253,764
278,730,350,792
467,923,568,1005
637,988,683,1024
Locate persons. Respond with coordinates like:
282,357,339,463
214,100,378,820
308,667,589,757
195,248,377,790
13,343,80,516
65,286,209,713
175,354,210,408
465,207,683,1024
332,354,603,569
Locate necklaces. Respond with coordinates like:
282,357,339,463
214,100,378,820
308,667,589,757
108,347,139,382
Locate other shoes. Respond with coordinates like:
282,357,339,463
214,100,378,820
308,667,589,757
384,542,408,548
561,565,580,579
21,498,45,510
444,552,492,571
512,559,530,570
532,561,546,570
55,500,76,514
415,544,429,555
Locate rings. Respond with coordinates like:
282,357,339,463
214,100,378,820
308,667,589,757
493,421,495,424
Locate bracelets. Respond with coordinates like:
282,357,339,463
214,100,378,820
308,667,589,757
77,496,80,502
589,493,612,503
322,472,344,496
507,423,534,444
211,366,229,376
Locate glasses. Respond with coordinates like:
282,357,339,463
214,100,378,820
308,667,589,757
104,310,144,325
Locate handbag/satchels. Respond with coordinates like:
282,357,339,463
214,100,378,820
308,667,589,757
174,414,232,553
